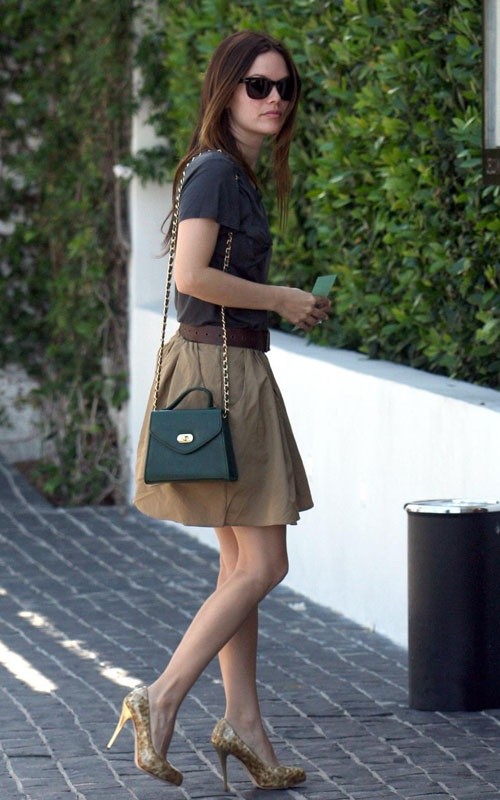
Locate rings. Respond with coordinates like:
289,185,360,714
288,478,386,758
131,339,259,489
317,319,323,324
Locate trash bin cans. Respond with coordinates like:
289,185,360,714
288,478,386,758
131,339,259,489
403,498,500,712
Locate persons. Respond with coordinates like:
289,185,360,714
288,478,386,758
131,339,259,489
105,30,332,791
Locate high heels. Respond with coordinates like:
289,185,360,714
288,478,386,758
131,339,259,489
106,686,184,787
211,719,306,790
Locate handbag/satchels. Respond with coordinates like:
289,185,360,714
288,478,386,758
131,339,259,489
143,388,239,481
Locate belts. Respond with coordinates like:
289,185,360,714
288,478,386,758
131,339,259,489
179,324,271,352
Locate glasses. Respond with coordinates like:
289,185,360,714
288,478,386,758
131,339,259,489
239,74,297,100
